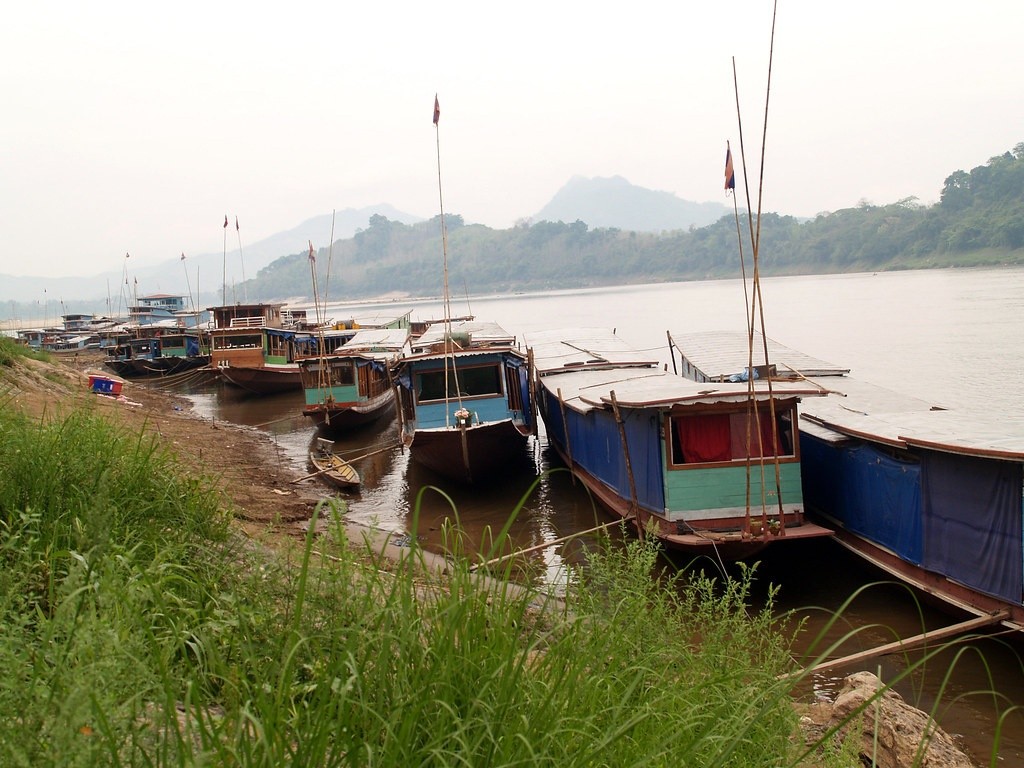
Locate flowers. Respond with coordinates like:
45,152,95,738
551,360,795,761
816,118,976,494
454,408,472,419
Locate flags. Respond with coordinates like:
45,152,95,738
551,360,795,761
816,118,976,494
224,215,227,228
433,98,440,123
235,216,239,231
309,245,315,261
181,252,184,260
725,150,734,189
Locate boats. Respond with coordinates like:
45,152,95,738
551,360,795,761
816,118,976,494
13,0,539,489
663,326,1023,639
530,0,837,546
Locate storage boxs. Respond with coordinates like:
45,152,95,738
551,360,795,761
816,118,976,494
88,375,123,395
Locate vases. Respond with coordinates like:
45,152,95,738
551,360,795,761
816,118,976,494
457,418,471,428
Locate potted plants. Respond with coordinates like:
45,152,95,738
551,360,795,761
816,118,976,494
767,519,781,536
750,518,762,536
